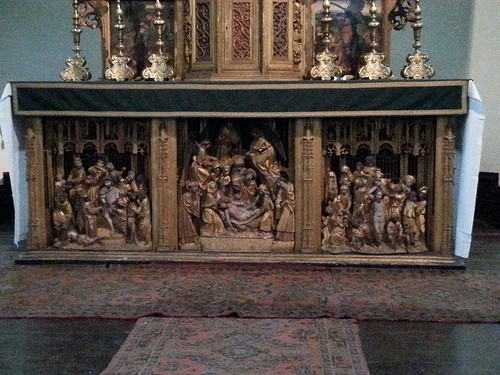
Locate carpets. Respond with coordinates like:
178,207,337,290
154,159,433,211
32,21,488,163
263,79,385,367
99,317,370,375
0,258,500,323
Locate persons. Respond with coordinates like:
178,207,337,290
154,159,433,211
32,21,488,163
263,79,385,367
36,136,434,257
